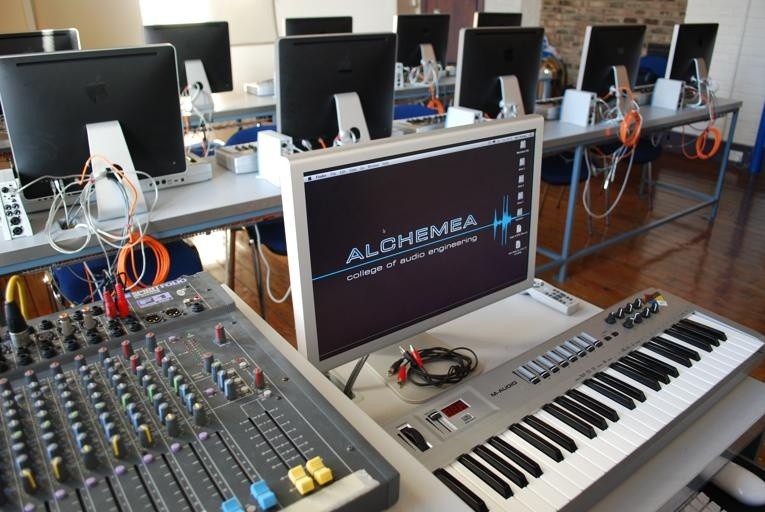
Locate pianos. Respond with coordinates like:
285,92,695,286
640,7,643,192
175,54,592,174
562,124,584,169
382,285,765,512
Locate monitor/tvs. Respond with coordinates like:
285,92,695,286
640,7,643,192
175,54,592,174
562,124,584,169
274,32,397,153
0,42,213,222
397,14,450,70
0,28,81,54
664,22,719,107
285,16,353,35
279,113,545,404
576,24,647,116
453,26,545,120
143,22,233,96
473,11,522,27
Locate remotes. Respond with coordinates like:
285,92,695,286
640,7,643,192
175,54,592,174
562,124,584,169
519,279,580,315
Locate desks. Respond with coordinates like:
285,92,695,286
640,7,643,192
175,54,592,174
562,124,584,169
0,64,743,322
